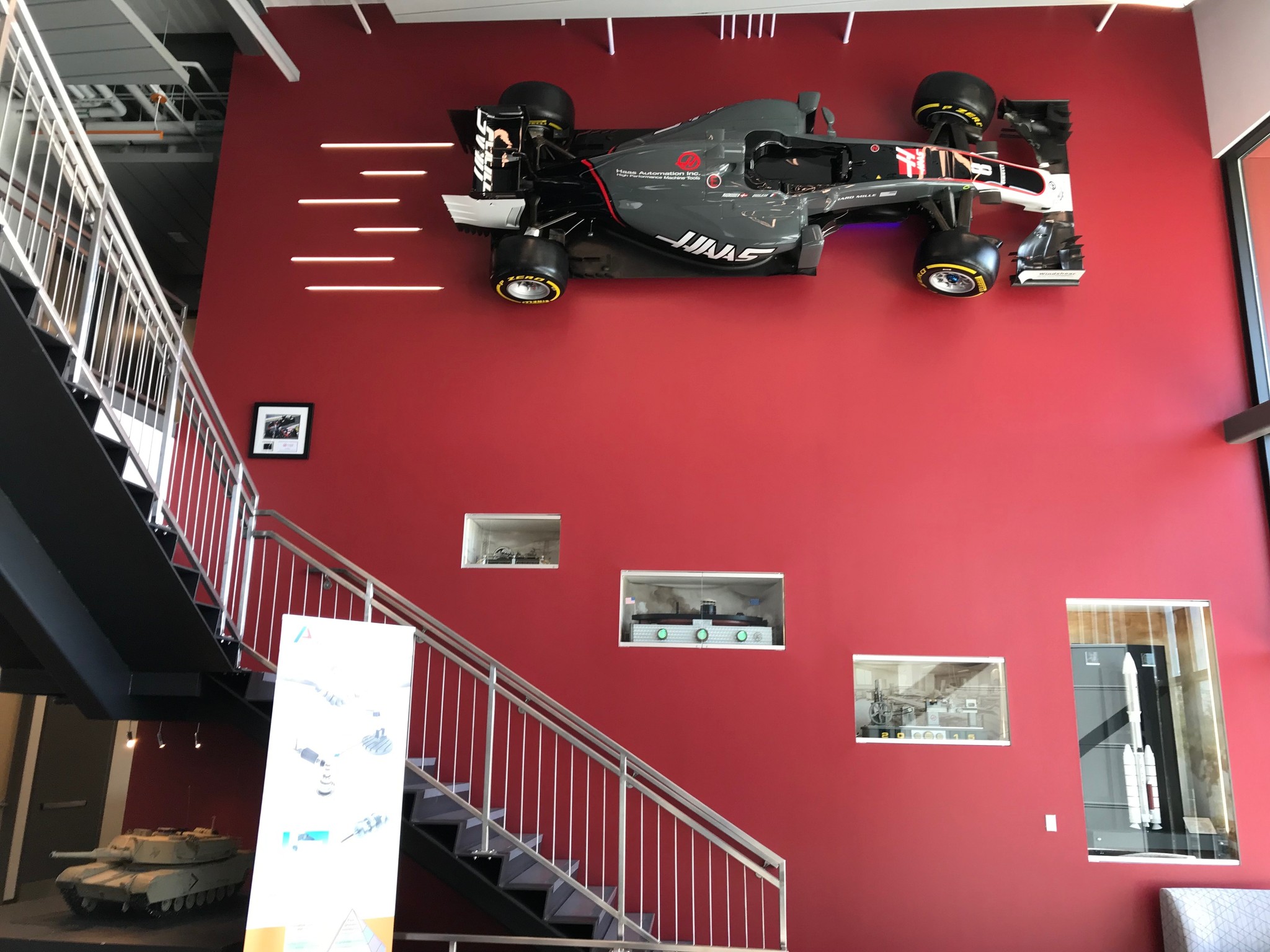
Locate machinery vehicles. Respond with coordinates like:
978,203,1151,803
50,815,252,922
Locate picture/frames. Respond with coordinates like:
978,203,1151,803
247,402,316,460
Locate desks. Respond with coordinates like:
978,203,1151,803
0,894,252,952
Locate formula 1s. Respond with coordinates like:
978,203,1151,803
439,81,1090,303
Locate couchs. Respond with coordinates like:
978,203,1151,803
1160,888,1270,952
